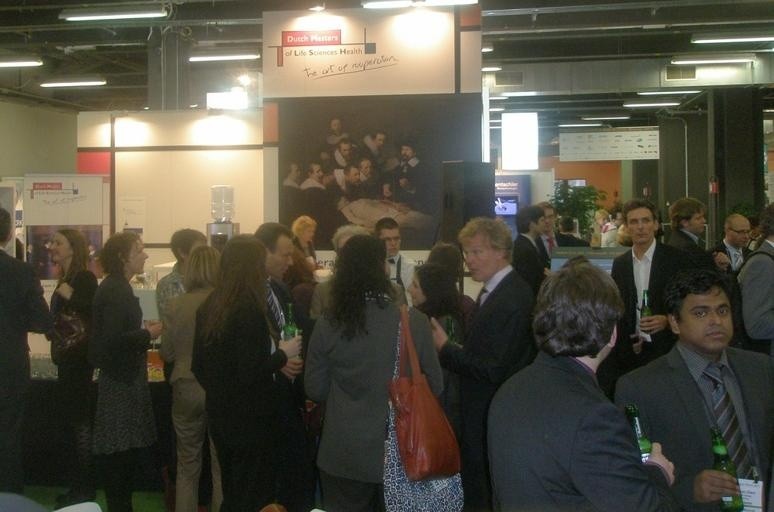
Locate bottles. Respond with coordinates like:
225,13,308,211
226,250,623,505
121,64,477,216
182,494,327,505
445,317,457,344
708,424,744,512
282,302,298,353
641,288,652,334
624,403,652,465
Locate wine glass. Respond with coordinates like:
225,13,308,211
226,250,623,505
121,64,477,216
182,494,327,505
144,319,164,353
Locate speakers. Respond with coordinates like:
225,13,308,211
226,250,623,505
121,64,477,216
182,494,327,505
440,159,495,249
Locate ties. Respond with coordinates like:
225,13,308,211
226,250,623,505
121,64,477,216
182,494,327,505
468,288,487,333
547,234,555,253
264,281,281,329
705,364,753,478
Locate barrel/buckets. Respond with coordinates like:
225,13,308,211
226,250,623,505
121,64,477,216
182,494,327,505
208,184,235,224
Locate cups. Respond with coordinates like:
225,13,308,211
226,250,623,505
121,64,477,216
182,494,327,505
313,269,332,283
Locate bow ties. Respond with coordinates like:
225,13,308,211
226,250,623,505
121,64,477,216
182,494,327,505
388,259,395,264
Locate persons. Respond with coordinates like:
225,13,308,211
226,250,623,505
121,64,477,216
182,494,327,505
0,207,53,495
613,266,774,511
599,198,774,383
43,229,97,508
85,229,162,511
25,242,36,264
156,199,593,510
278,108,437,249
86,243,100,262
488,256,674,511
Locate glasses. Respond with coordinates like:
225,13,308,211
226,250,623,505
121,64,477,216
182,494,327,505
728,227,751,233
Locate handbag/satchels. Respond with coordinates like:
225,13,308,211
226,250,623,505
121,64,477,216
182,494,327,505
50,311,85,351
388,374,461,482
384,400,464,512
